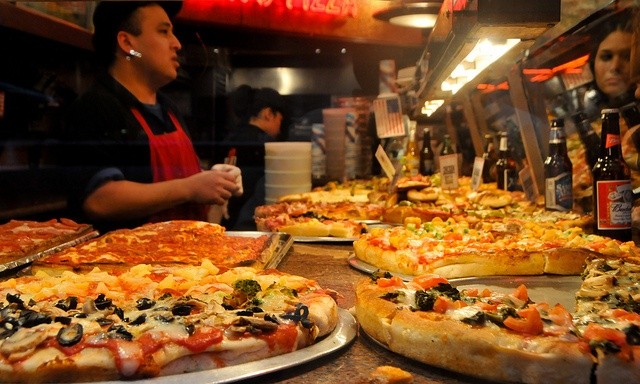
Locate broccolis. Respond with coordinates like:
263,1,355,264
236,278,261,309
414,283,457,315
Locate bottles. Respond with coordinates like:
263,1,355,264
592,108,633,242
420,128,436,175
544,118,573,211
439,134,454,155
496,131,516,191
403,126,420,173
482,134,498,183
619,101,640,155
571,111,601,171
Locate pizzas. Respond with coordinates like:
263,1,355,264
0,258,345,384
253,196,384,238
352,216,640,279
34,220,280,269
0,218,93,263
356,253,640,383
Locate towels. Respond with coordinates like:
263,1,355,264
205,156,244,227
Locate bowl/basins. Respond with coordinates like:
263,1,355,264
263,140,313,207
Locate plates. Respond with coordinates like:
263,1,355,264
224,231,296,271
0,220,100,272
94,304,357,384
294,218,381,244
346,247,417,281
361,276,588,384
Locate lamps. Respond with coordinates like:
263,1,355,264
372,0,443,28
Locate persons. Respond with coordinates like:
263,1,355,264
47,1,245,233
589,14,640,107
216,84,283,230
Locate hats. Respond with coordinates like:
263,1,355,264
92,0,183,32
